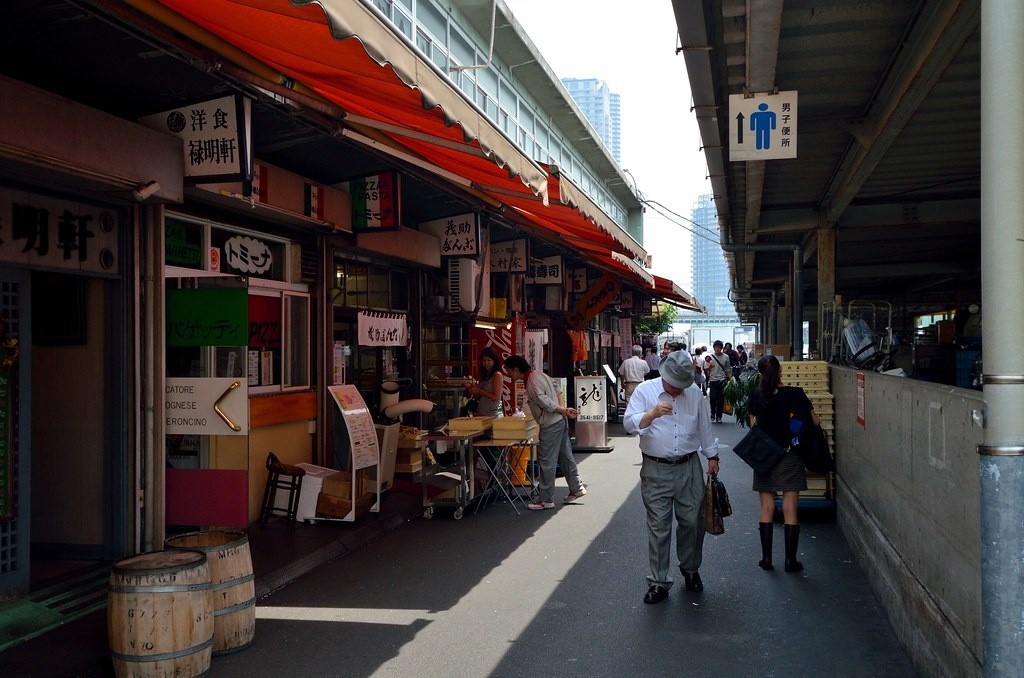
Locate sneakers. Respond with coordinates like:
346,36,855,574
563,486,586,502
528,500,554,510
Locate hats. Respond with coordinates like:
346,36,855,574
658,350,696,389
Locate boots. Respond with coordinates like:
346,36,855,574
783,523,803,571
758,522,774,570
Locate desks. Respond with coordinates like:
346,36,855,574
470,438,540,515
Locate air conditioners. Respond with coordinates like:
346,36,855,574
448,258,490,317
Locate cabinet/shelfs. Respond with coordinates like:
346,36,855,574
420,428,509,520
424,337,477,388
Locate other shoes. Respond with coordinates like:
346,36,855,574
711,413,722,423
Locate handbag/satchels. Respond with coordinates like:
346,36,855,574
459,391,480,417
703,472,733,535
733,425,785,477
723,398,733,416
791,385,833,476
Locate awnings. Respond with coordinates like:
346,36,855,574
123,0,707,313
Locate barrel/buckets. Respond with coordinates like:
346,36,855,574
164,528,255,656
108,549,215,678
503,445,529,485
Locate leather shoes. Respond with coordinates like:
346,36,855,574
643,585,669,603
679,568,704,591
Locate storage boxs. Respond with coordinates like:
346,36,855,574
764,344,834,496
271,463,372,517
448,416,538,439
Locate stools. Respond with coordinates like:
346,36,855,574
258,452,305,532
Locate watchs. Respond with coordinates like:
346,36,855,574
709,456,719,461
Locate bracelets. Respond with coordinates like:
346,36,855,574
479,388,481,392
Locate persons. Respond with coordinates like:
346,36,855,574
504,355,588,509
618,340,754,423
464,346,503,496
746,355,819,572
624,351,719,603
